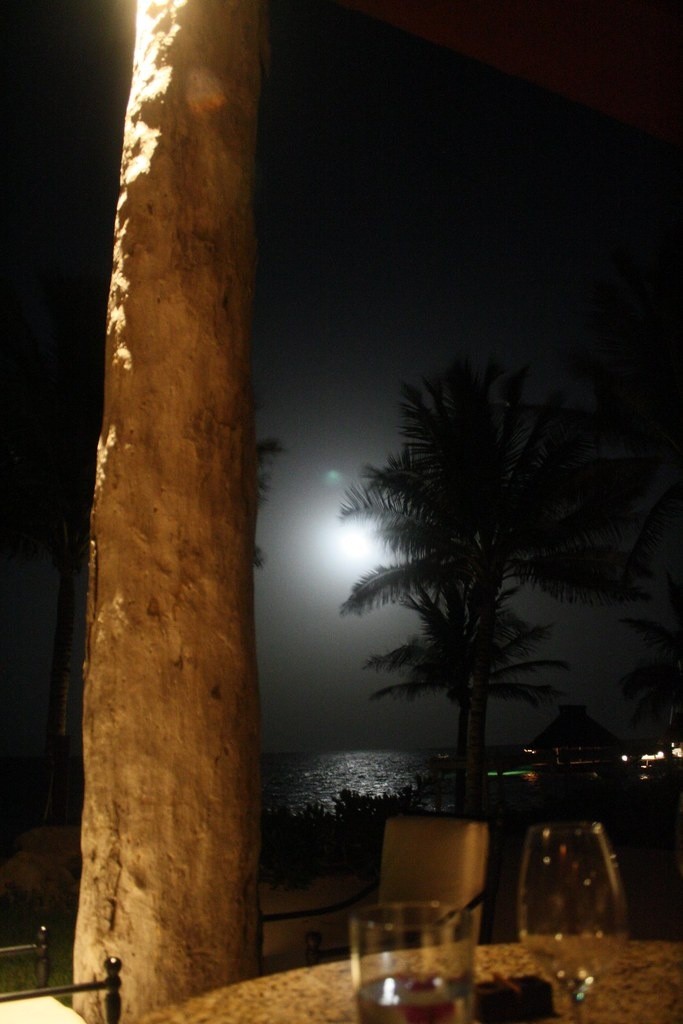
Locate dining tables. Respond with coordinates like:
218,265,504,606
141,940,683,1024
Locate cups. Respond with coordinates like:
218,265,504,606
350,900,475,1024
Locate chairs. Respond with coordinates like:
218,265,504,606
1,925,123,1024
371,817,488,945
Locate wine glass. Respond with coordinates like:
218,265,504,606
516,818,629,1024
